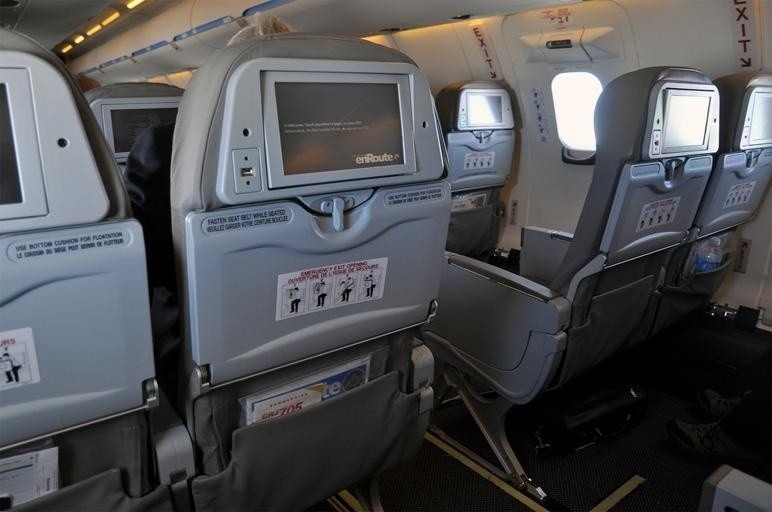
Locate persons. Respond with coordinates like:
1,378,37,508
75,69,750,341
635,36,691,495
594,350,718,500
316,282,327,307
290,287,301,313
123,13,297,383
365,274,376,298
726,190,751,206
466,159,491,168
3,352,21,383
341,277,354,302
640,207,674,229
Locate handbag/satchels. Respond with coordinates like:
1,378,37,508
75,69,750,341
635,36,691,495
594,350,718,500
536,381,652,455
733,306,760,333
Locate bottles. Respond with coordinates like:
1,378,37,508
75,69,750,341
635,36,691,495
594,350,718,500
693,236,723,274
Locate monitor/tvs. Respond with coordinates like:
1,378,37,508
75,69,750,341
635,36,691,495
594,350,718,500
749,92,772,145
467,93,505,126
661,90,717,152
260,71,417,189
101,103,179,158
0,68,49,221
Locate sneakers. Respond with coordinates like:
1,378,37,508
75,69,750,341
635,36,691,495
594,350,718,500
668,415,769,468
696,386,754,419
